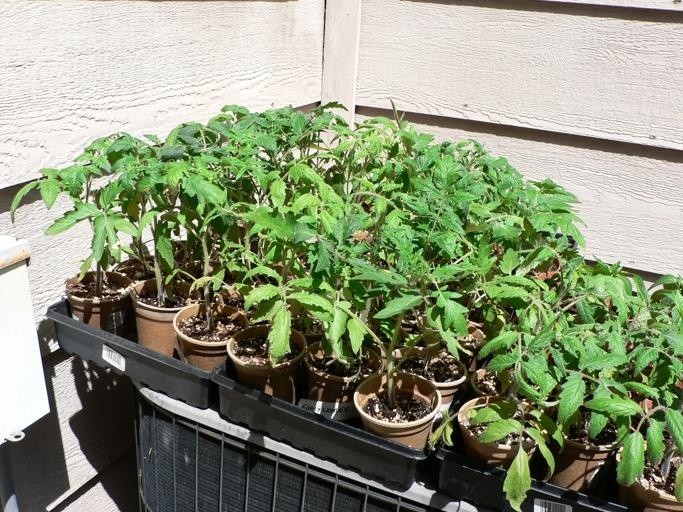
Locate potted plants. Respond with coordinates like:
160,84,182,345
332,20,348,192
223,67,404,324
43,106,683,509
